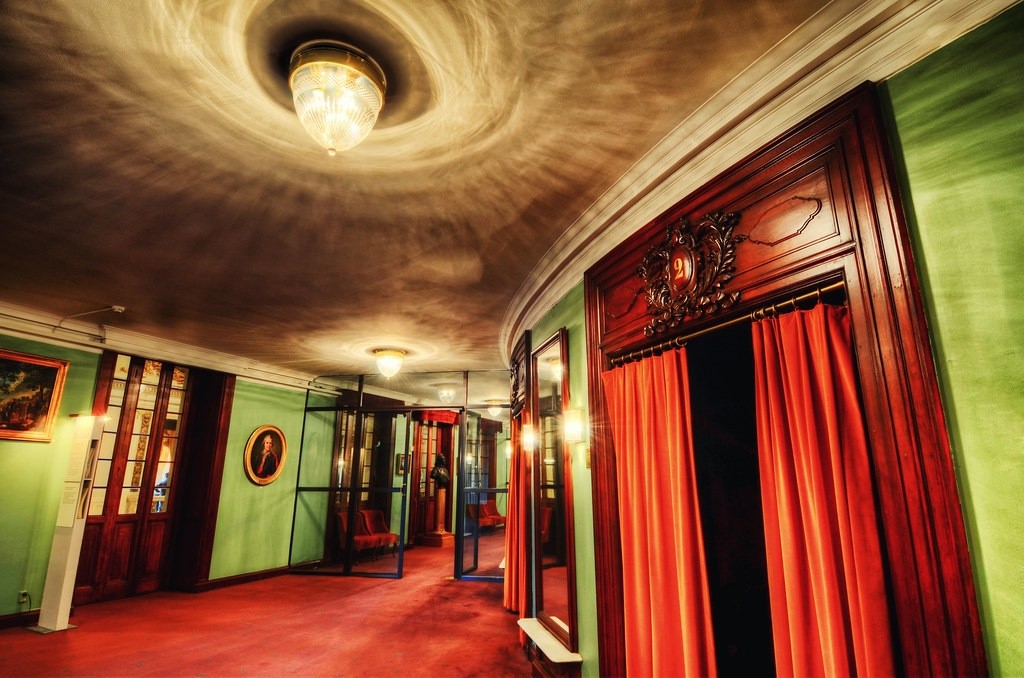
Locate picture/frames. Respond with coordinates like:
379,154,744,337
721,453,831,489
244,425,288,485
0,348,70,442
397,453,411,475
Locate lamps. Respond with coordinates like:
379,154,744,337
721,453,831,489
372,348,406,378
288,35,387,156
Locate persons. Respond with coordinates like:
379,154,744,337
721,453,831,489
155,473,169,512
430,454,451,488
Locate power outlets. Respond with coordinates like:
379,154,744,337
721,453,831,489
18,591,27,602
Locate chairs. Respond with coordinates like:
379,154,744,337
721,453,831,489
466,499,506,538
336,509,399,565
